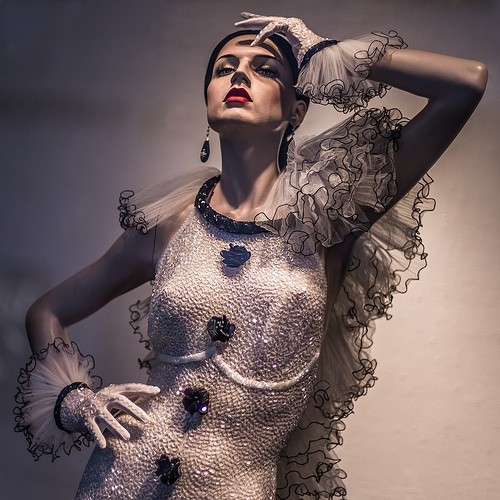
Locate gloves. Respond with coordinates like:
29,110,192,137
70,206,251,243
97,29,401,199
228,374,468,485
14,336,161,463
233,10,409,115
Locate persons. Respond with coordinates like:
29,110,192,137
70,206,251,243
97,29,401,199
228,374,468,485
23,10,488,499
